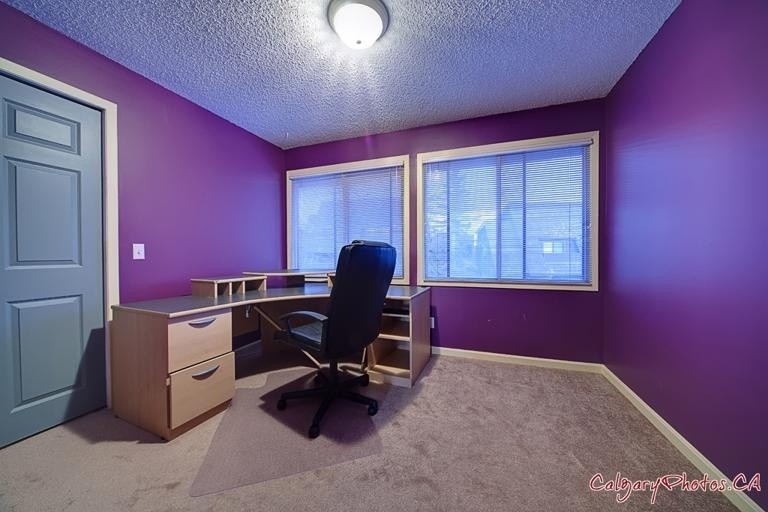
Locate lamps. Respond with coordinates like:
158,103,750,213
326,0,389,51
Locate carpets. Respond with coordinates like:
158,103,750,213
188,366,384,497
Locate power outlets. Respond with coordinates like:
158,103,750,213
430,317,435,329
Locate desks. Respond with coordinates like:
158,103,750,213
109,270,431,442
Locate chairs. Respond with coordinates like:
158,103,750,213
271,238,397,438
